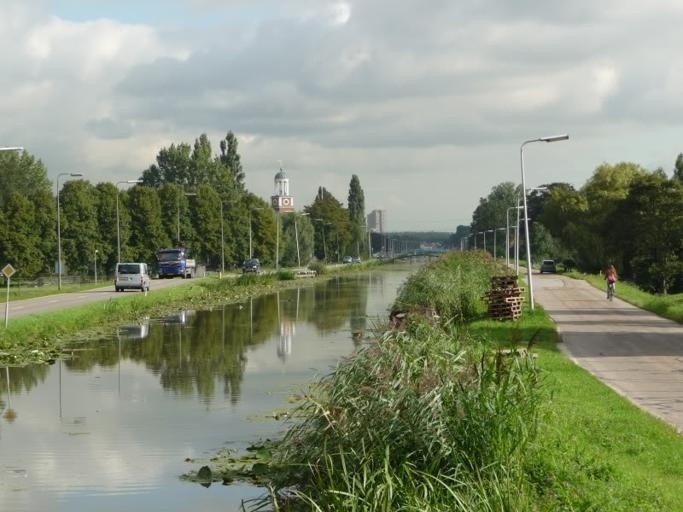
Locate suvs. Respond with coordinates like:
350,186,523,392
116,313,150,341
241,258,260,274
114,263,150,292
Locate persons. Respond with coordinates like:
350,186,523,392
604,264,619,298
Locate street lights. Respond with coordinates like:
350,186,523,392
0,146,23,153
249,207,264,258
115,179,144,263
506,205,524,270
57,360,83,427
520,134,570,317
116,341,144,404
459,218,531,270
275,210,295,270
515,187,547,287
218,198,237,274
294,212,309,269
57,172,83,291
176,192,197,250
311,212,410,268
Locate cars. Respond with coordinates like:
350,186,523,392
539,259,557,274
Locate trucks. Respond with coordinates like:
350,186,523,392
160,309,196,328
154,248,196,279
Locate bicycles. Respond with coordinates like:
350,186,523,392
604,276,618,303
